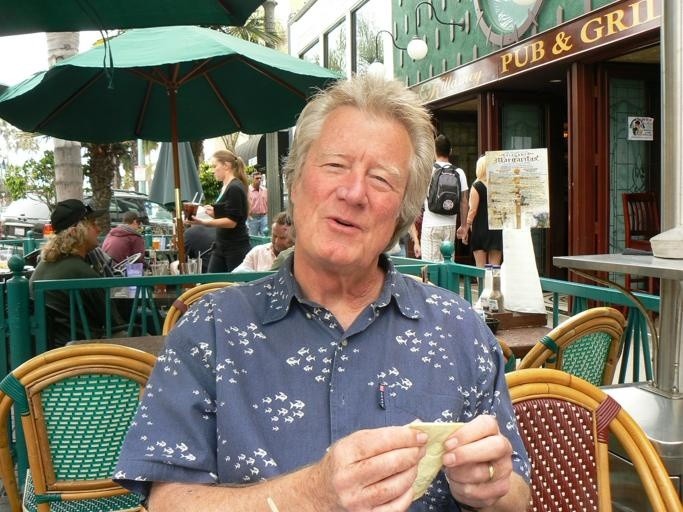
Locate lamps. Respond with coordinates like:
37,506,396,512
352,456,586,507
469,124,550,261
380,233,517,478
366,30,407,78
407,2,464,60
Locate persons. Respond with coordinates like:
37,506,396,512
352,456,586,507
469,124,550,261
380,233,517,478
389,134,502,281
28,199,128,346
179,147,296,275
114,69,532,512
103,211,150,270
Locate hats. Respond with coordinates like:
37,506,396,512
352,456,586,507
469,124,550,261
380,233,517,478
50,199,106,234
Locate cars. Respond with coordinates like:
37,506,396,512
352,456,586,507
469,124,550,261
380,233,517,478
83,190,175,236
0,197,53,248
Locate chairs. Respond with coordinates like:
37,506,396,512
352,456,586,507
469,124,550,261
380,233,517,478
0,343,157,512
495,339,515,372
515,306,626,386
505,368,682,512
4,243,240,359
623,192,660,325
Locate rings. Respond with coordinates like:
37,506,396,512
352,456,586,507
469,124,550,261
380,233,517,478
487,463,495,483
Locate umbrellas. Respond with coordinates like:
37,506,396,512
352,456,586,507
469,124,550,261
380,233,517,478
2,2,261,34
148,141,205,206
0,26,346,273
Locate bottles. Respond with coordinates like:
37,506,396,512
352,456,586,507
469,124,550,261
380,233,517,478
489,267,504,312
481,264,493,312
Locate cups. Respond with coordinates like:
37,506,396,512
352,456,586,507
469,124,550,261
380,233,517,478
197,206,206,218
183,202,198,221
126,258,203,293
152,238,161,250
161,238,165,250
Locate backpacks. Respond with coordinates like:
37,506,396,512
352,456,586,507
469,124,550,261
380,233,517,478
426,163,461,215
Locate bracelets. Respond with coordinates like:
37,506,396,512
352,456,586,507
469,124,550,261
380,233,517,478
262,481,281,512
